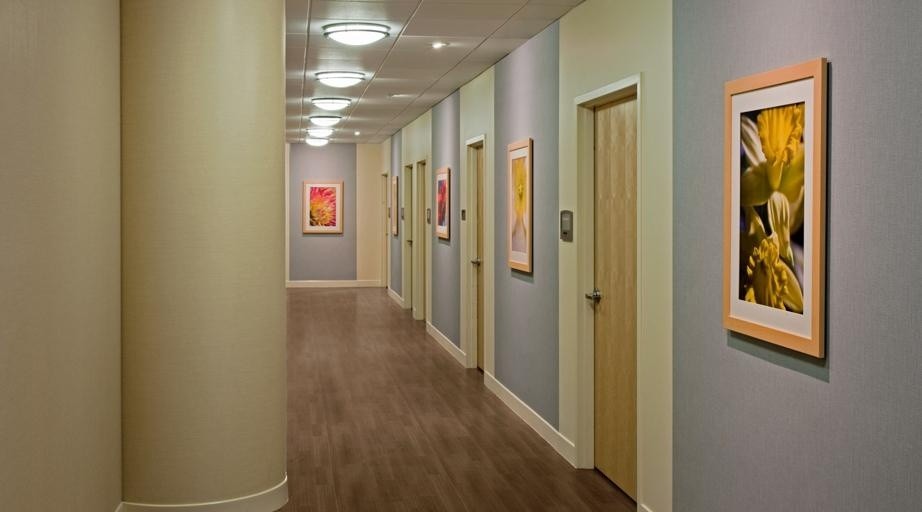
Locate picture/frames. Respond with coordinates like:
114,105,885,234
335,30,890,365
505,137,534,275
724,55,825,357
434,168,449,240
391,175,399,236
302,179,343,234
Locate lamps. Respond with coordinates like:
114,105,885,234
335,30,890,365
304,138,328,146
310,98,351,110
309,116,342,125
306,128,334,138
313,72,364,89
321,20,391,45
